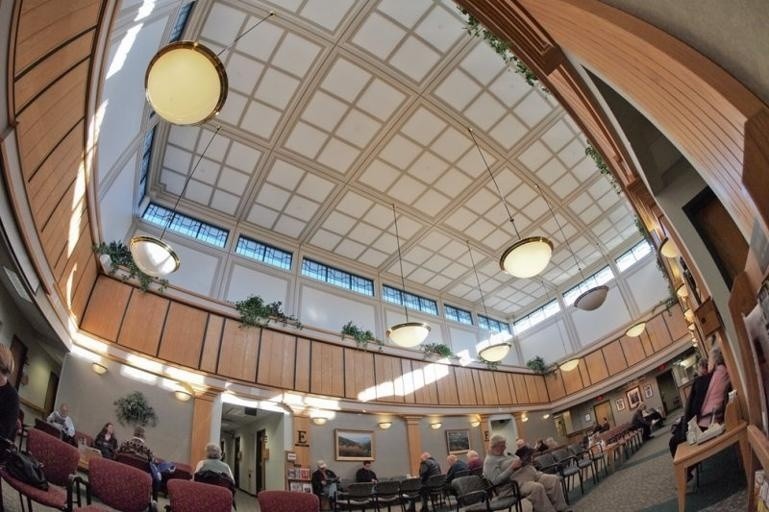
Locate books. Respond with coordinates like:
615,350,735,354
289,466,313,493
323,475,341,484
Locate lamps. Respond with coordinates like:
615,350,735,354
469,128,554,280
541,281,580,371
385,205,432,351
466,242,511,363
145,11,273,127
91,362,108,376
129,126,221,278
536,184,610,311
658,239,698,348
598,245,647,338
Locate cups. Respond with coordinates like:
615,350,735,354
685,430,697,447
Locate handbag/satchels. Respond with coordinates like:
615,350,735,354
1,438,49,492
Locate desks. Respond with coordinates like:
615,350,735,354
672,419,752,512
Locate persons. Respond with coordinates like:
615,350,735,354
312,459,341,509
642,406,666,428
670,342,730,483
482,434,574,512
445,435,560,483
46,404,75,440
668,359,710,485
188,441,237,486
95,422,119,458
631,402,655,440
0,343,22,459
405,451,442,512
592,421,602,433
354,460,378,484
114,424,158,467
601,417,610,431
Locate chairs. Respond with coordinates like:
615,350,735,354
747,424,768,511
0,384,644,512
696,382,732,489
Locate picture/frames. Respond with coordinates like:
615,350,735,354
613,384,655,412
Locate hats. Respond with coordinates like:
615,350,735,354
316,460,326,469
